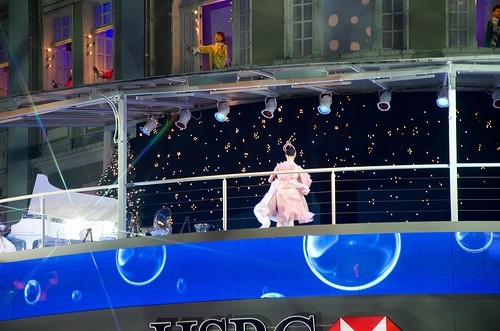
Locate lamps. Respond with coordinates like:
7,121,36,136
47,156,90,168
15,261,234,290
214,100,230,122
140,116,158,136
377,89,390,111
175,108,191,129
318,92,332,114
261,96,277,118
437,85,449,107
492,90,500,108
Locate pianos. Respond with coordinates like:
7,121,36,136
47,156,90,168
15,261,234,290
4,173,118,250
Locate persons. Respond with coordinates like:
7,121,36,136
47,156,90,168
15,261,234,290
62,68,72,88
92,66,113,79
485,4,500,49
190,31,230,70
253,141,315,228
